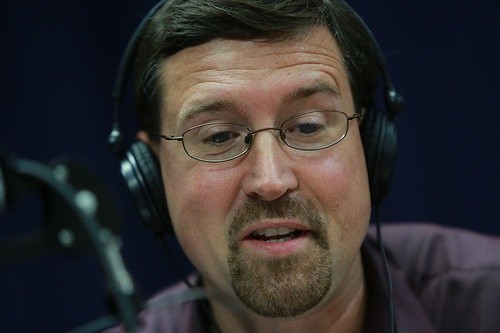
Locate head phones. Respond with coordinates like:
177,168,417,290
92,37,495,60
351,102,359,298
105,1,404,242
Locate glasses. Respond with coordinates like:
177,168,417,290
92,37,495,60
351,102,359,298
151,108,364,163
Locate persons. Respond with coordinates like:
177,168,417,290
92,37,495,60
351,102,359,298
98,0,500,333
